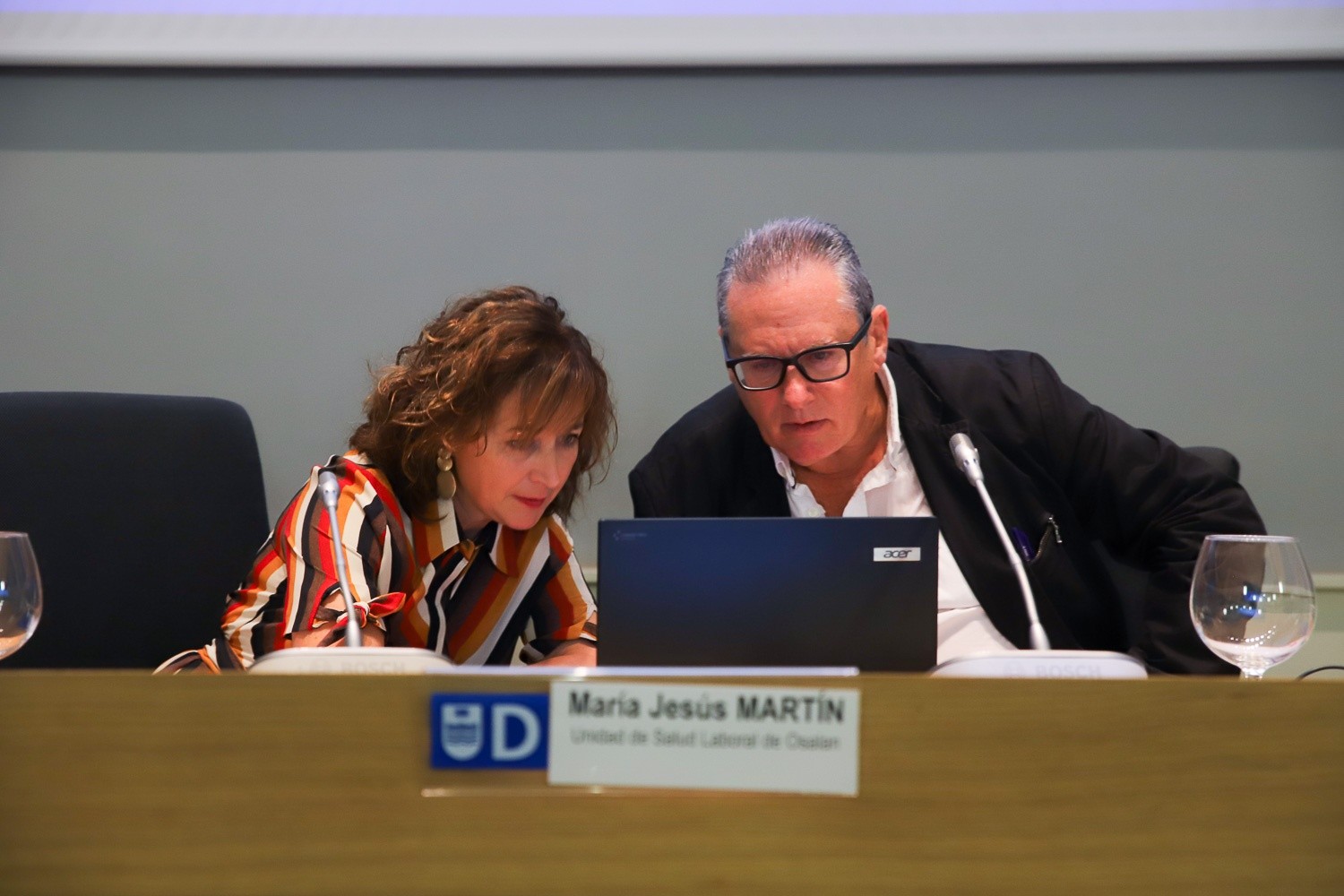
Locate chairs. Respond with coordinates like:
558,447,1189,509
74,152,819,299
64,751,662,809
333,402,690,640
0,386,268,671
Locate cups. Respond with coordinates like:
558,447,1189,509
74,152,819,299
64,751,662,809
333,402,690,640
0,531,43,659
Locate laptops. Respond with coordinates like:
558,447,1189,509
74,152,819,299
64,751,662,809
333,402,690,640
594,516,938,675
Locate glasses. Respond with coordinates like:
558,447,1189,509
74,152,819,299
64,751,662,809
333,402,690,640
722,315,872,391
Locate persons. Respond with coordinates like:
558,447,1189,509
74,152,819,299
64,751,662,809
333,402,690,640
152,286,620,676
627,218,1269,677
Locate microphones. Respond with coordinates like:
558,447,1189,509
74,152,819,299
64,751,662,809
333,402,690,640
926,432,1149,678
247,472,455,674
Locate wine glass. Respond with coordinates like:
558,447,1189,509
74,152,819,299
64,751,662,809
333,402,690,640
1189,534,1317,678
